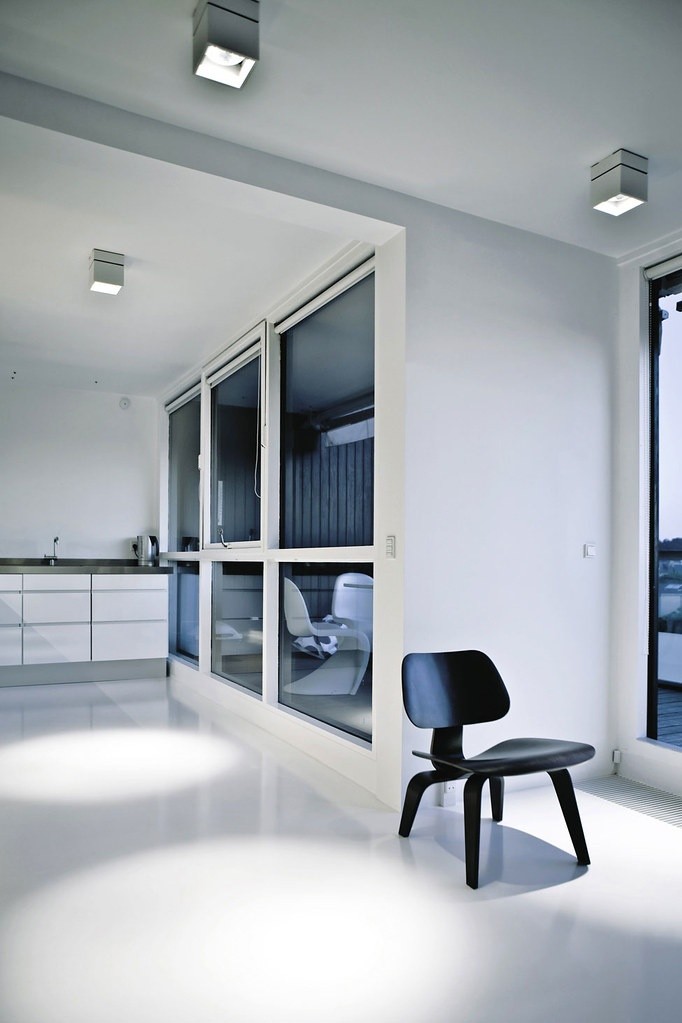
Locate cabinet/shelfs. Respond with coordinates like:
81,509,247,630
178,565,348,671
0,564,174,685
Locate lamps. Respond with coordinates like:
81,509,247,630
88,247,124,295
193,0,259,89
590,147,649,215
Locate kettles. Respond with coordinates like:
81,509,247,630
137,536,159,567
180,537,199,566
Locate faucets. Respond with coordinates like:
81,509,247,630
44,536,60,566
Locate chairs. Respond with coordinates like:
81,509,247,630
283,577,371,695
397,650,596,892
320,573,373,655
